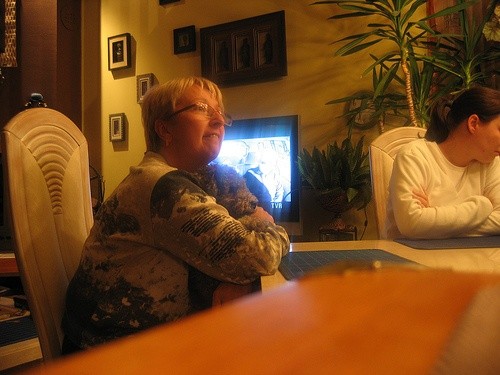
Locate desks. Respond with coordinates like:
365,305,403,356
261,234,500,292
23,266,500,375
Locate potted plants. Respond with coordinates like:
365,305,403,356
295,134,372,241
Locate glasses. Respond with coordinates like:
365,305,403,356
174,102,232,127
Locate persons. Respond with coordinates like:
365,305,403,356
384,76,500,240
243,151,284,202
61,75,290,357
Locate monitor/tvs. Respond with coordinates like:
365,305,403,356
211,113,305,235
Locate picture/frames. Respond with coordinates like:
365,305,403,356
109,113,124,142
108,33,130,71
173,25,196,54
136,73,154,103
200,10,288,87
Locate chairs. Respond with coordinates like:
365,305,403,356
0,107,93,363
368,127,426,239
